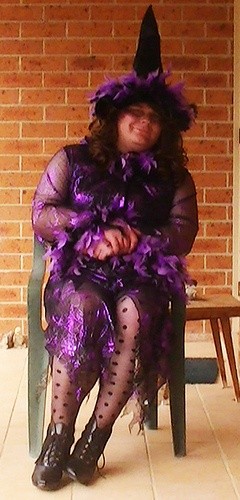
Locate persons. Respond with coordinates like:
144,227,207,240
30,77,200,492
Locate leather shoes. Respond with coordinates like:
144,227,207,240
31,423,75,491
64,414,112,486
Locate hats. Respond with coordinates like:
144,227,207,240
85,5,196,131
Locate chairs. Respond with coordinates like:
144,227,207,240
27,237,185,457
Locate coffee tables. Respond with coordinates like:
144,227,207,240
159,293,240,404
